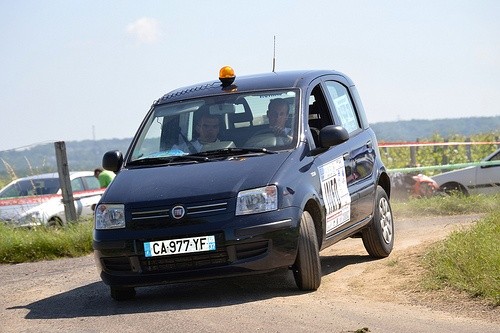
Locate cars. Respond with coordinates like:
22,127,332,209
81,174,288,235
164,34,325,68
429,147,500,198
0,170,116,227
92,68,394,302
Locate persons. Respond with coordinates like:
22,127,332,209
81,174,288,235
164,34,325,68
171,111,222,153
19,190,28,196
93,168,117,189
257,98,294,147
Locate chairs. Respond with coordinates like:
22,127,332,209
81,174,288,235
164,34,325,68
192,97,326,149
